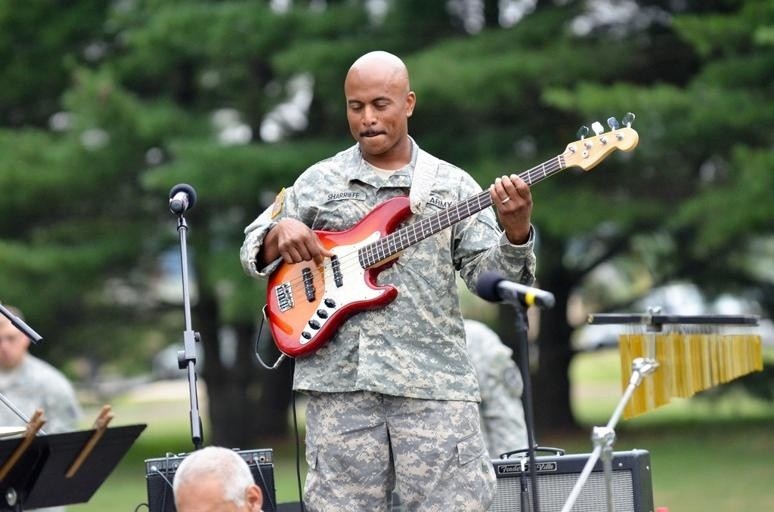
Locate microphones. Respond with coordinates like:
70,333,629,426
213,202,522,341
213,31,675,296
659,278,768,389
168,183,197,216
476,272,555,311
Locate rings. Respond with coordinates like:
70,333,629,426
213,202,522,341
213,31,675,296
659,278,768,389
500,195,510,205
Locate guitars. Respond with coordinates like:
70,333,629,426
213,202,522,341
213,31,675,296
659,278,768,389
266,113,638,359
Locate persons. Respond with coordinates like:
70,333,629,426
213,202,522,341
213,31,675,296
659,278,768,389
170,444,266,512
458,318,530,462
0,303,88,435
239,50,538,512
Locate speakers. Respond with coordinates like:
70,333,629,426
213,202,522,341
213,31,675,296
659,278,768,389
492,448,655,512
